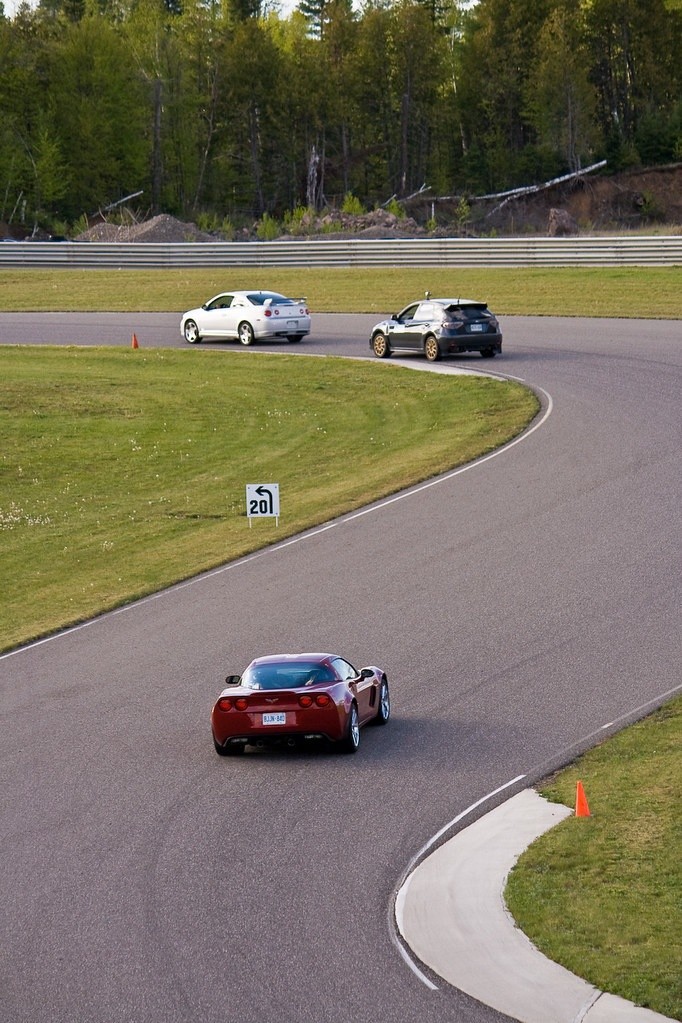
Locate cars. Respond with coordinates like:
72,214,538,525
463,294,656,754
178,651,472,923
179,290,312,347
368,298,503,363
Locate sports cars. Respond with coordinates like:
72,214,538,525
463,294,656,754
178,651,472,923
210,651,391,756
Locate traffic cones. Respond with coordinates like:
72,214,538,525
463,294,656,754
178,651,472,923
575,778,592,817
131,333,138,350
